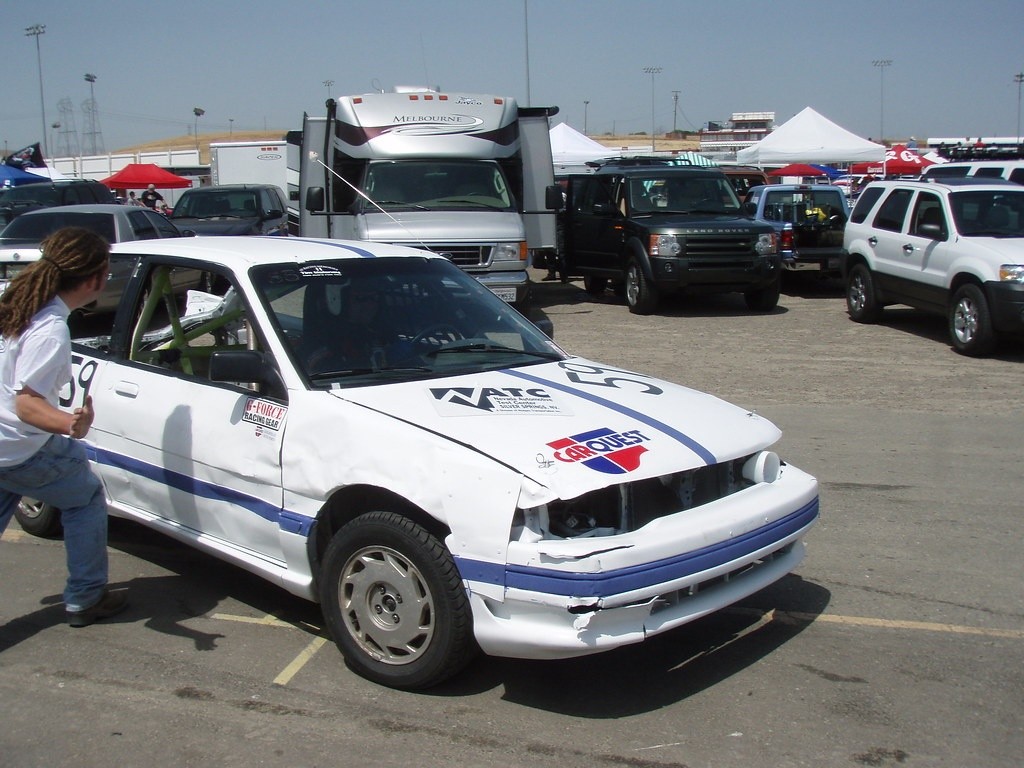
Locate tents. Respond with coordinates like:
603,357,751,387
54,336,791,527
737,106,886,209
99,164,193,193
847,143,950,197
549,122,621,172
667,150,719,168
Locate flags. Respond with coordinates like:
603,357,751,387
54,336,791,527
6,143,47,170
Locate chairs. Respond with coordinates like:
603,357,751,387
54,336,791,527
200,198,231,215
983,204,1010,230
304,266,413,356
244,199,255,211
921,206,943,229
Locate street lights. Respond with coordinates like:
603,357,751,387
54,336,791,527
872,59,893,141
24,23,46,158
643,66,663,152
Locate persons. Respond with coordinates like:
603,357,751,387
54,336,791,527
0,227,129,625
906,137,917,148
540,191,568,283
139,184,165,210
127,191,139,206
956,137,986,148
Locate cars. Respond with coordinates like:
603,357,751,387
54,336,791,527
717,136,1024,294
1,85,560,340
13,236,821,691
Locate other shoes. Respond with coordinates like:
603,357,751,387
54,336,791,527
561,279,569,284
542,275,555,280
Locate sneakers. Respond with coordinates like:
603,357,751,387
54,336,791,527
70,590,129,625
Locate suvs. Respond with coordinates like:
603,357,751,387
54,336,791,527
840,172,1024,357
529,155,782,316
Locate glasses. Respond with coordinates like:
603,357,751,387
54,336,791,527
102,269,112,280
